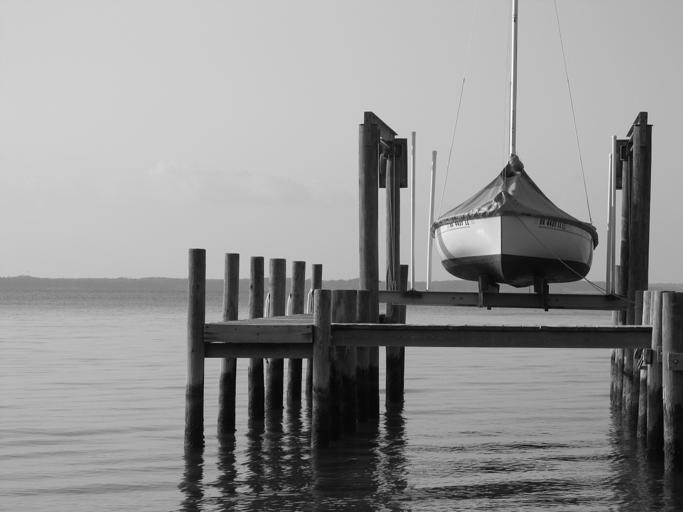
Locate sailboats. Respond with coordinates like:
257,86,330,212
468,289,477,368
432,1,600,289
